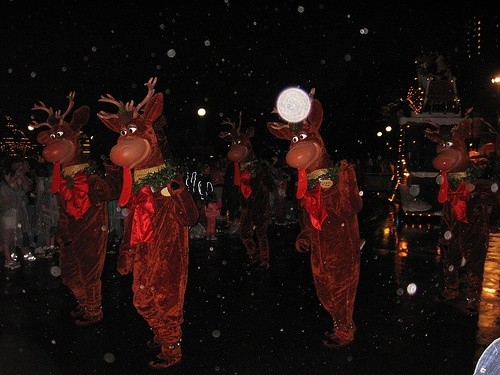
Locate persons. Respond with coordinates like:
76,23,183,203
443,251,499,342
1,160,124,268
181,159,299,242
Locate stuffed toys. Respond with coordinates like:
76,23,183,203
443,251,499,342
218,111,272,271
266,87,361,349
25,91,117,326
94,76,202,368
426,129,482,226
